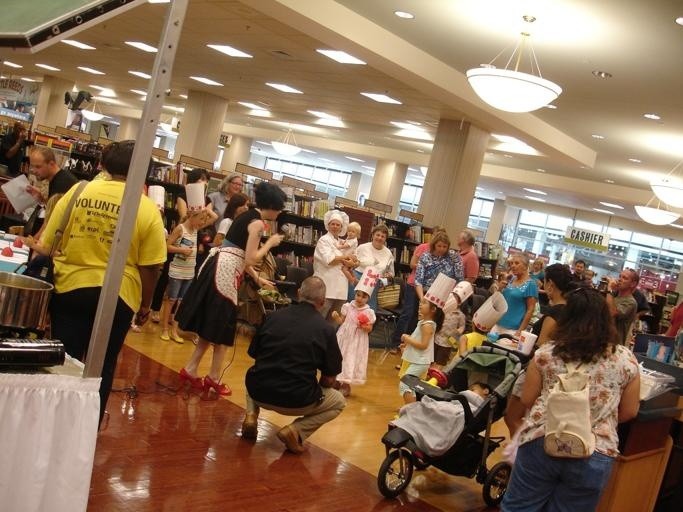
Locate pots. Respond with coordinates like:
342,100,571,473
0,271,55,337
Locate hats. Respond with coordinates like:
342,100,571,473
322,208,349,239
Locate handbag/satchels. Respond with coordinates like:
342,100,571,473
374,276,404,311
13,255,55,283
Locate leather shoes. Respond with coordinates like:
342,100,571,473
156,329,187,345
240,412,260,439
275,424,306,455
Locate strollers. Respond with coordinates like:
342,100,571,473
376,333,541,504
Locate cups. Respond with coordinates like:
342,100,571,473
518,331,537,355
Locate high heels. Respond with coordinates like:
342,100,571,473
199,371,234,399
178,365,204,392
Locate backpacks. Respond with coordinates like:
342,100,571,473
543,357,598,461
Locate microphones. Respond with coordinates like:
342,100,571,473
269,224,289,252
506,274,513,285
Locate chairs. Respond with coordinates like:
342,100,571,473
237,265,492,351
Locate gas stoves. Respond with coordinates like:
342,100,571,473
0,330,66,371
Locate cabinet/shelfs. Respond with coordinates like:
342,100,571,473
146,177,497,280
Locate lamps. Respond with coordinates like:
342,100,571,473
81,95,104,122
634,195,681,225
466,15,563,113
160,111,180,136
649,156,683,209
271,128,302,155
